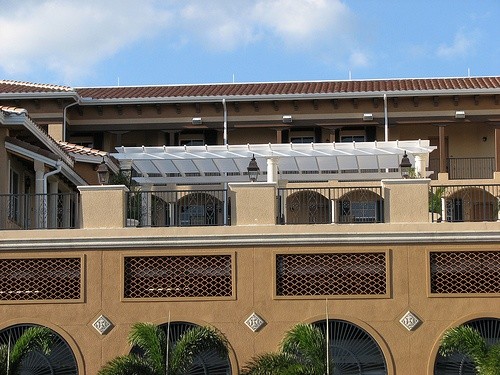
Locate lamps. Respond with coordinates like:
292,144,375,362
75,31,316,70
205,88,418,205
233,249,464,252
96,157,112,185
398,150,413,179
245,154,261,182
